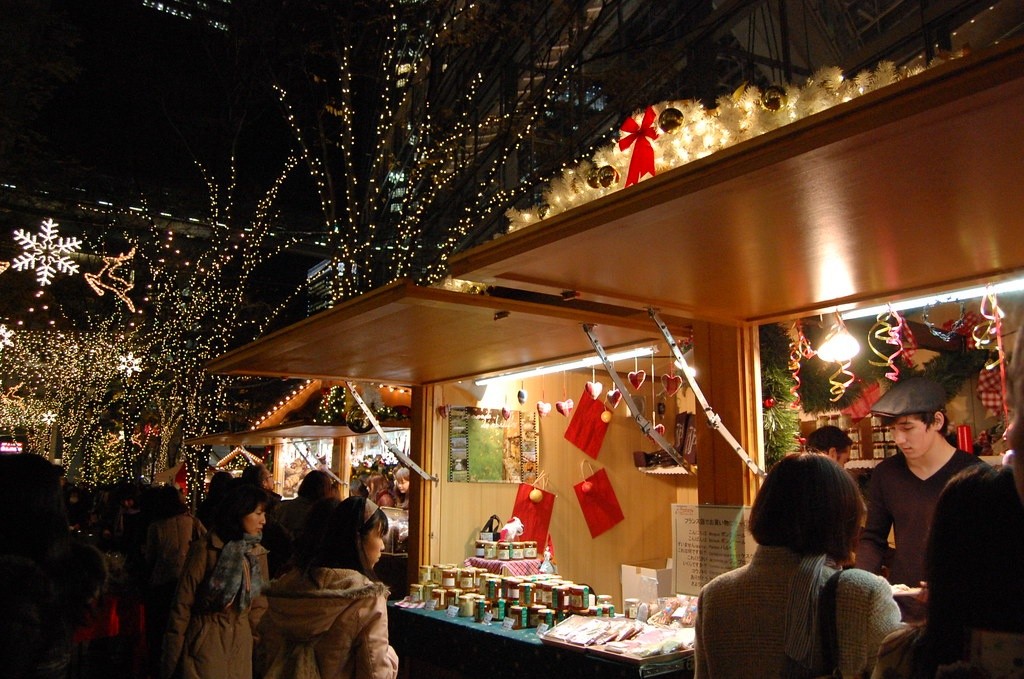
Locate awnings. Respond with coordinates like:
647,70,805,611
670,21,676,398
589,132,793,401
203,277,695,481
183,420,410,484
445,34,1024,474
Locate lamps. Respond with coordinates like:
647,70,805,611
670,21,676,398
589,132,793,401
839,270,1024,325
471,341,662,387
816,306,860,366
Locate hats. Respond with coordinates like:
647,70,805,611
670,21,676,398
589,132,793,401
870,377,946,419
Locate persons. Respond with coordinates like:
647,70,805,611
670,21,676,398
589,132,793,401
694,452,906,679
1003,297,1024,499
854,377,988,621
808,425,853,468
869,461,1024,679
0,448,413,679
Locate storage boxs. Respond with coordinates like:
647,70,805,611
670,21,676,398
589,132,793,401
619,558,675,614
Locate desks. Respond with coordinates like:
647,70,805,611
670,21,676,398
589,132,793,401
388,599,702,679
464,557,541,576
